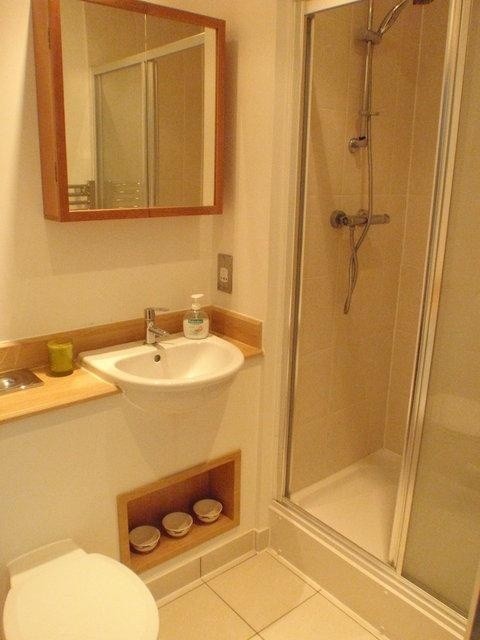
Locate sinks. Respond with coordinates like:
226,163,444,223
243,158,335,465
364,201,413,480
78,327,244,412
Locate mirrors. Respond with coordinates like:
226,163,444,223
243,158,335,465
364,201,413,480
31,0,230,223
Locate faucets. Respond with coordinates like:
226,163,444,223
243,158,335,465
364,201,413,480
144,306,170,343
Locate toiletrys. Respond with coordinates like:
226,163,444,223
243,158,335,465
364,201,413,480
183,293,209,340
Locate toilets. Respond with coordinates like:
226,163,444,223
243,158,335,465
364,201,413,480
2,541,162,639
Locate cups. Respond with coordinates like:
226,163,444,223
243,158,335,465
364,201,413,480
48,339,74,376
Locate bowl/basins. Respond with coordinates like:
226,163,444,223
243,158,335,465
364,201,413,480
163,511,193,538
129,525,161,553
193,498,223,523
